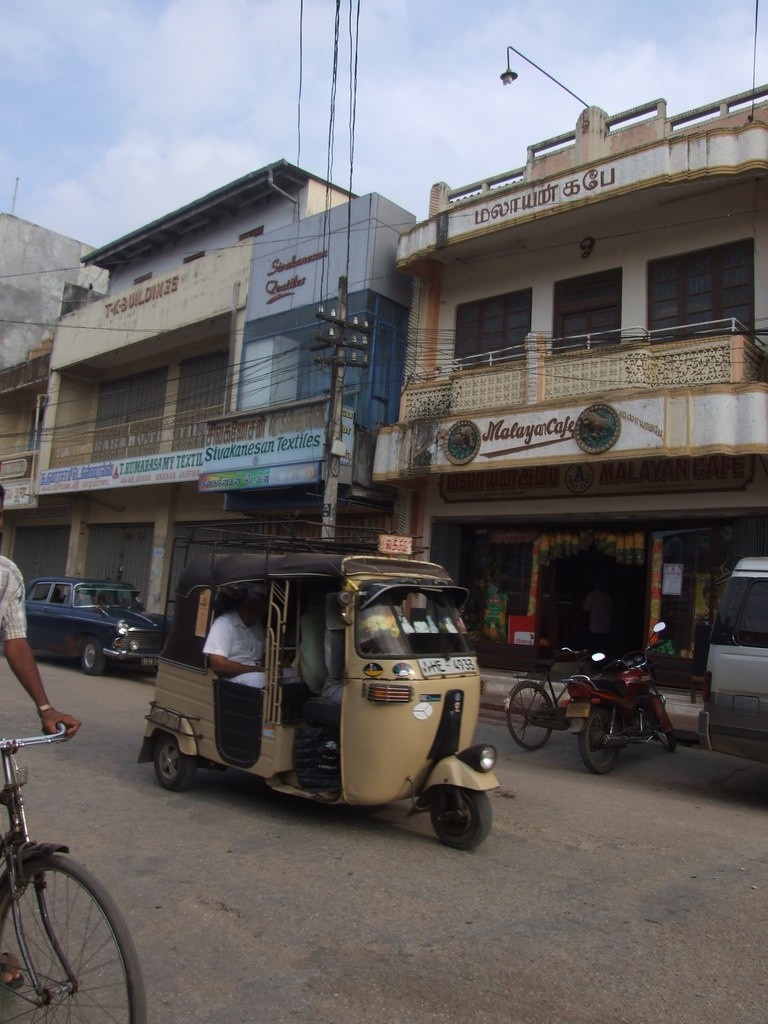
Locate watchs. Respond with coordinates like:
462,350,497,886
36,703,52,716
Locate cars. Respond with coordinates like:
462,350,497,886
23,576,174,675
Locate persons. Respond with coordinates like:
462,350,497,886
582,575,616,675
695,582,721,625
202,581,302,689
0,485,83,993
323,577,345,705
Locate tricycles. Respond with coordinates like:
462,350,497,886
136,517,501,851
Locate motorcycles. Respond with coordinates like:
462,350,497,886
560,622,676,773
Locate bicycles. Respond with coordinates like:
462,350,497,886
505,648,610,749
0,723,147,1024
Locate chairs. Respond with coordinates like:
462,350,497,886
299,604,339,729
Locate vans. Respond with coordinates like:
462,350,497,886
699,557,768,764
